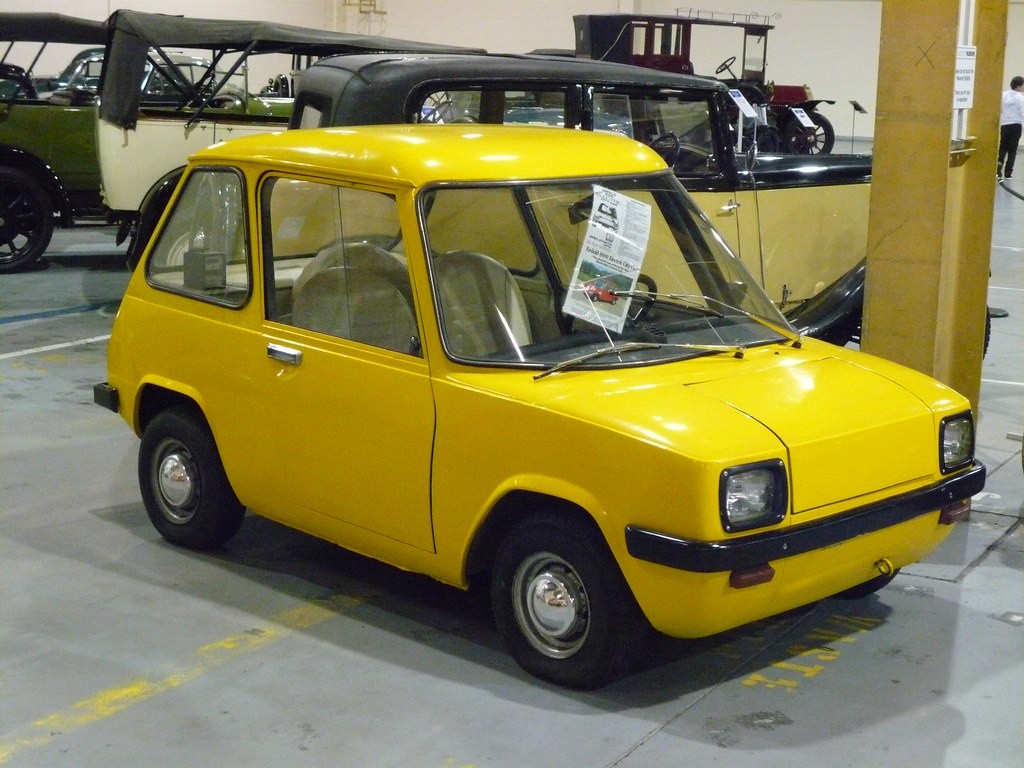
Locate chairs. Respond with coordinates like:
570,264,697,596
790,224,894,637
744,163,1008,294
292,242,534,363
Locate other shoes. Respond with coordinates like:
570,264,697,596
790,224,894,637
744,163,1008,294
1005,176,1012,179
997,162,1002,177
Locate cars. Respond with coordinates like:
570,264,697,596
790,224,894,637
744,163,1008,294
0,12,225,274
53,47,274,116
91,8,488,245
92,123,986,690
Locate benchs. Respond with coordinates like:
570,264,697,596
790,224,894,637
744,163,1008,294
634,54,694,75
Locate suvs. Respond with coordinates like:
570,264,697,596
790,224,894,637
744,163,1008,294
288,48,991,362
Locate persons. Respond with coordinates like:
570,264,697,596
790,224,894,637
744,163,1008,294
997,76,1024,178
